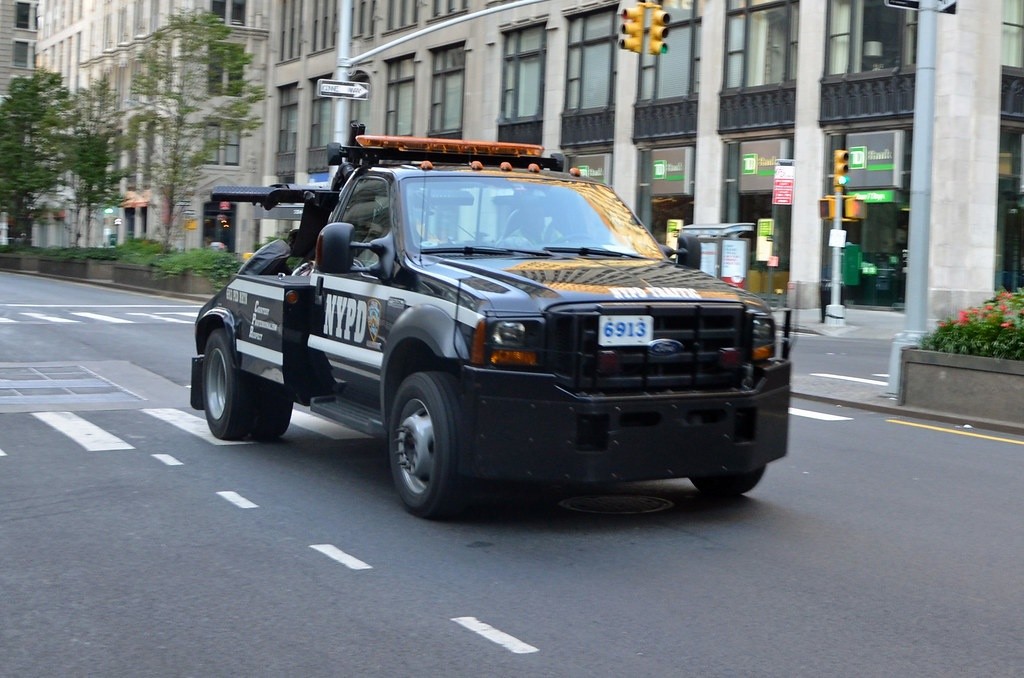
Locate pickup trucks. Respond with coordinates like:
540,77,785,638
189,136,792,523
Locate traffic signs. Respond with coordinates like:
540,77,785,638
317,79,369,101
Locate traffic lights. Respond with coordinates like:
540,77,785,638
845,198,867,220
818,198,835,220
648,8,671,57
832,149,850,186
618,4,645,53
